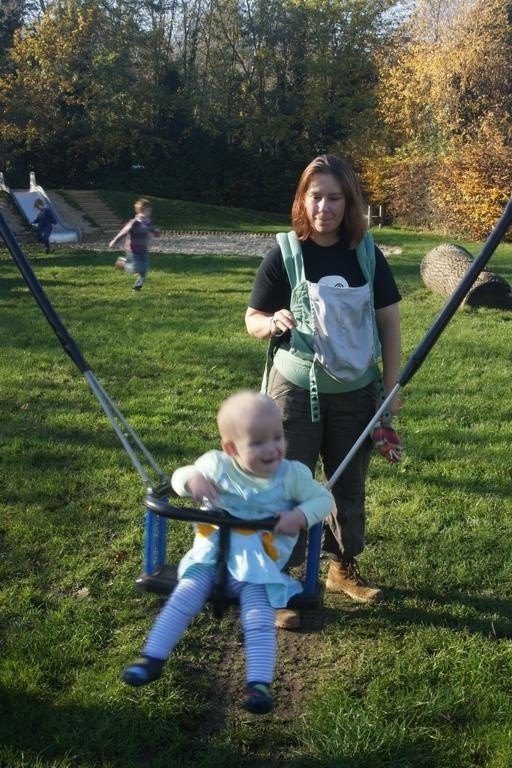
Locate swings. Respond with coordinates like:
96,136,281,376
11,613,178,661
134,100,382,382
0,183,512,631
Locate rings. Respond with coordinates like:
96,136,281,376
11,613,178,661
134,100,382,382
273,318,279,325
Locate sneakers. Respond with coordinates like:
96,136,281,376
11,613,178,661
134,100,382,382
122,655,163,687
245,684,274,715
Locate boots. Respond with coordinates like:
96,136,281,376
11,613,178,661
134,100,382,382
276,609,302,630
325,556,382,604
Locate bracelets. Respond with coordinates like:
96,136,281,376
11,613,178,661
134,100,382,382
269,317,285,337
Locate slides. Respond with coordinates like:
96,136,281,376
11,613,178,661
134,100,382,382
2,176,81,246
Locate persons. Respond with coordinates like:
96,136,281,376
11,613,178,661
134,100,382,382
108,198,161,293
30,198,58,252
121,388,336,715
245,155,404,631
